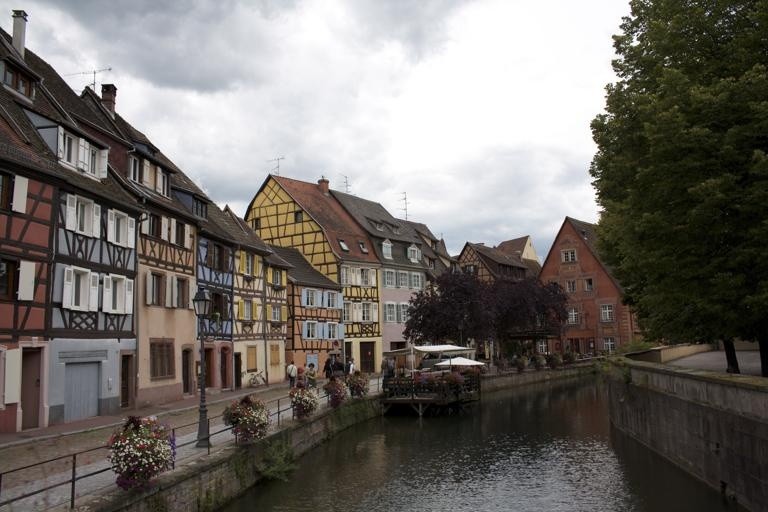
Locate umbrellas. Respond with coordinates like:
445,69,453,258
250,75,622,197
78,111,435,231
432,355,486,367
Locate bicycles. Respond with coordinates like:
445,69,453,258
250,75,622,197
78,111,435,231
249,369,266,387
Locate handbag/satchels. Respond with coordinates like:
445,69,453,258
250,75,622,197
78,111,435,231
288,374,291,377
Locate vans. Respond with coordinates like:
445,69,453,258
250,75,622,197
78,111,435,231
417,352,467,373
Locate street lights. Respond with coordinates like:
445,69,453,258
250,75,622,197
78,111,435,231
192,286,211,448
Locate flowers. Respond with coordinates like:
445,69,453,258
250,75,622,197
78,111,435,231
289,371,370,418
221,393,272,441
105,415,177,490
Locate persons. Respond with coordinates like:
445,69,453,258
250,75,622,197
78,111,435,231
305,363,317,388
321,357,332,384
286,361,297,389
379,356,397,377
296,364,306,388
332,356,344,377
416,363,422,369
343,358,356,385
